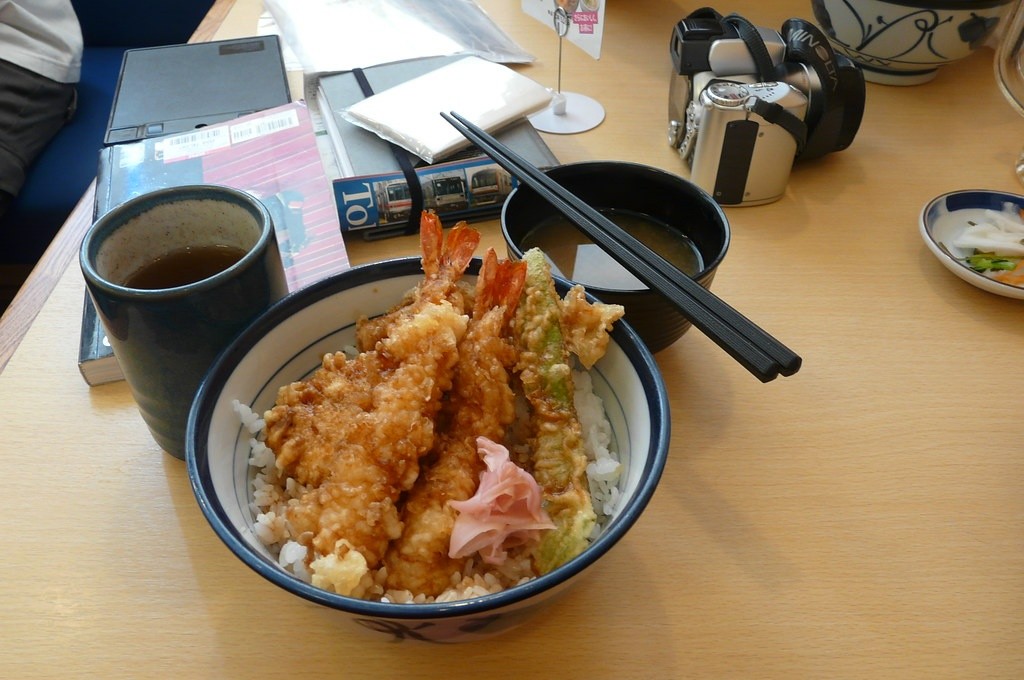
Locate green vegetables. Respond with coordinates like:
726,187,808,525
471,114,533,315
965,253,1016,272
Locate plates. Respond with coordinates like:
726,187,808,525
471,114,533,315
918,189,1024,301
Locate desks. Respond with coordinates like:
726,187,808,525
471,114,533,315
0,1,1024,680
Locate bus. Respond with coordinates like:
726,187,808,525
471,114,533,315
470,169,512,206
421,176,468,211
375,181,413,222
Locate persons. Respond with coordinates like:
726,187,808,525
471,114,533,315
0,0,83,319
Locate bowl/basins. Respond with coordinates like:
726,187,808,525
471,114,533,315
184,258,671,643
501,161,730,354
811,0,1016,86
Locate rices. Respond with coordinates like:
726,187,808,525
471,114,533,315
230,342,621,606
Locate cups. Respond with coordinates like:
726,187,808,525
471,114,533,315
80,184,289,461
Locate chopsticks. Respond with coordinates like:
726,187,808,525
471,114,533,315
439,111,802,383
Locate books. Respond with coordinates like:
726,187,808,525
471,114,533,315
316,54,561,185
78,98,352,389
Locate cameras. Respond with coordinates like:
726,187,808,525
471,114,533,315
667,10,867,207
